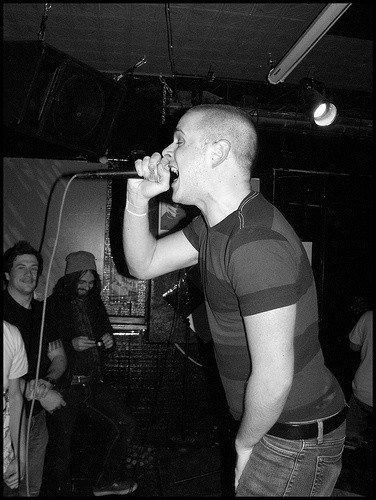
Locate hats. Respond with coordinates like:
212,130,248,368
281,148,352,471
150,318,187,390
65,251,97,275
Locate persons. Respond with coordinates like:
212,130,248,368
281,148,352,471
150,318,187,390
1,320,26,500
43,251,137,500
1,240,67,499
346,310,376,451
124,105,351,498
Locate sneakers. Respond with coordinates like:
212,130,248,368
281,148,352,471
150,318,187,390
345,437,359,449
93,477,137,496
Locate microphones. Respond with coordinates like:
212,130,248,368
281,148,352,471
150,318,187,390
75,166,143,179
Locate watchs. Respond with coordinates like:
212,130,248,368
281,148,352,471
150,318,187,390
43,376,57,388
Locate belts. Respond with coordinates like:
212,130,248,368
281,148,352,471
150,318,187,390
267,406,349,440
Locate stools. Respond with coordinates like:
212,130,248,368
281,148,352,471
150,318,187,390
57,420,98,496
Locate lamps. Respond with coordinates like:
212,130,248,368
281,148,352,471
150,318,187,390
301,85,338,129
